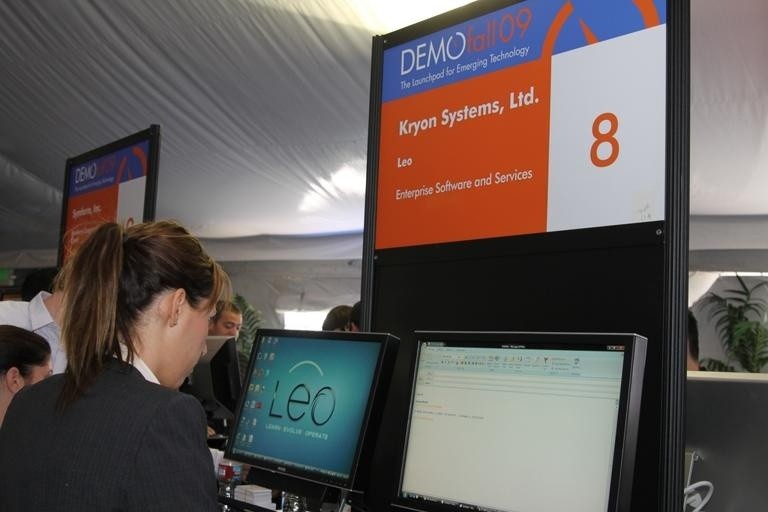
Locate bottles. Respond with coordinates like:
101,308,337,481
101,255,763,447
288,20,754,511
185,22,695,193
217,458,244,512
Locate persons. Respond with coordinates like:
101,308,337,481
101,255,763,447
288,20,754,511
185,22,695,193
0,217,235,512
0,271,67,378
0,324,54,429
209,299,250,392
686,309,701,371
322,304,352,332
350,300,360,331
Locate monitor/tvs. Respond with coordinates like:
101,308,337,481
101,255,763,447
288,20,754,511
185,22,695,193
386,328,650,512
222,327,401,512
190,335,242,436
